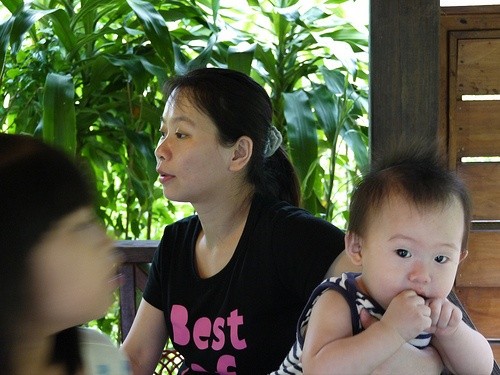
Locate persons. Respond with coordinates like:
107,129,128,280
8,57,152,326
119,68,446,375
269,160,494,375
0,133,134,375
